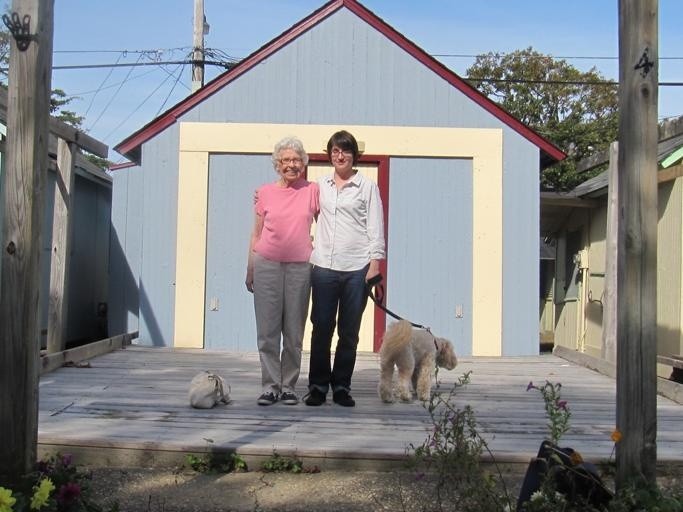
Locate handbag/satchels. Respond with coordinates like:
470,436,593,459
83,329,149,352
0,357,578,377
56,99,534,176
190,372,231,408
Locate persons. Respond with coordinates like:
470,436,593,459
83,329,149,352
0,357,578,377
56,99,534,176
253,129,388,407
242,136,321,406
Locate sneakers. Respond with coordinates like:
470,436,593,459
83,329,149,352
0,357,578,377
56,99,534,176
281,392,298,404
334,390,355,406
302,388,326,406
257,392,278,404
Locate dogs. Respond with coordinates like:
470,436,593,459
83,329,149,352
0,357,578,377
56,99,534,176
377,318,459,403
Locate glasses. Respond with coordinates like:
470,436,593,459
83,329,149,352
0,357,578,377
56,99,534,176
277,158,301,164
